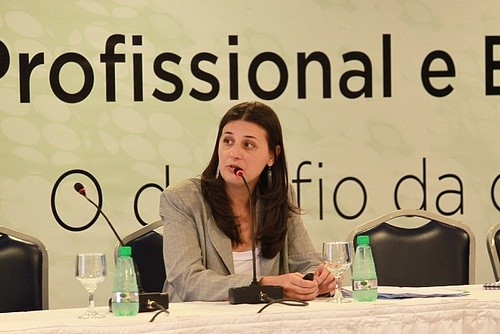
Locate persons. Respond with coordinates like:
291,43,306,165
158,100,338,304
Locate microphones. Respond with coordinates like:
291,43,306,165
229,168,283,306
74,182,169,313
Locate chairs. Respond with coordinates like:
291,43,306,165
485,222,500,284
344,209,478,289
0,225,50,314
113,218,169,296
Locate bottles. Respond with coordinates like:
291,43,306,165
112,246,140,317
351,236,378,302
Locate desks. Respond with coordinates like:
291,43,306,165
0,282,500,334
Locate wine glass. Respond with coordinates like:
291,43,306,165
75,253,107,319
322,242,352,304
